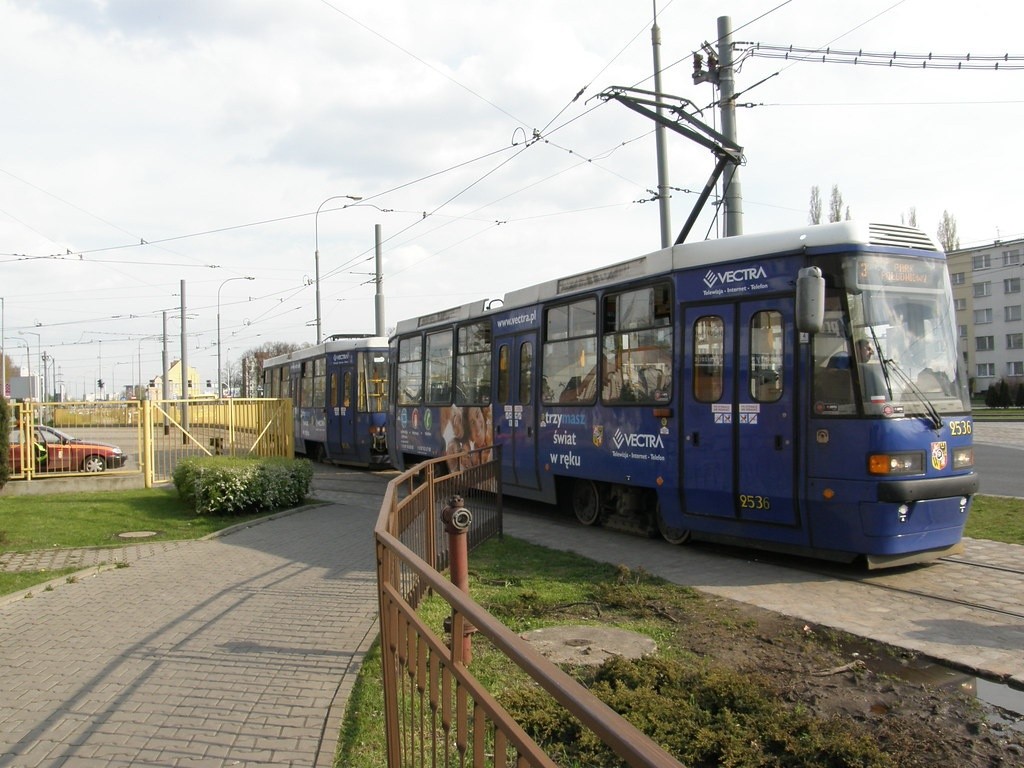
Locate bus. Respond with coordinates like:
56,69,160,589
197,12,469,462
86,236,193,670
262,333,400,470
390,91,981,574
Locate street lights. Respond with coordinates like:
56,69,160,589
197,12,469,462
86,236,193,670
315,193,364,345
216,276,256,400
19,331,43,426
4,336,33,404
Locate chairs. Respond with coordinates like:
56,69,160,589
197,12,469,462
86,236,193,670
827,351,850,369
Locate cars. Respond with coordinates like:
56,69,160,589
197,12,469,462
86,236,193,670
9,424,130,473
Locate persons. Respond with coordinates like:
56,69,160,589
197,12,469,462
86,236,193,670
436,403,496,475
854,339,875,368
34,434,49,465
